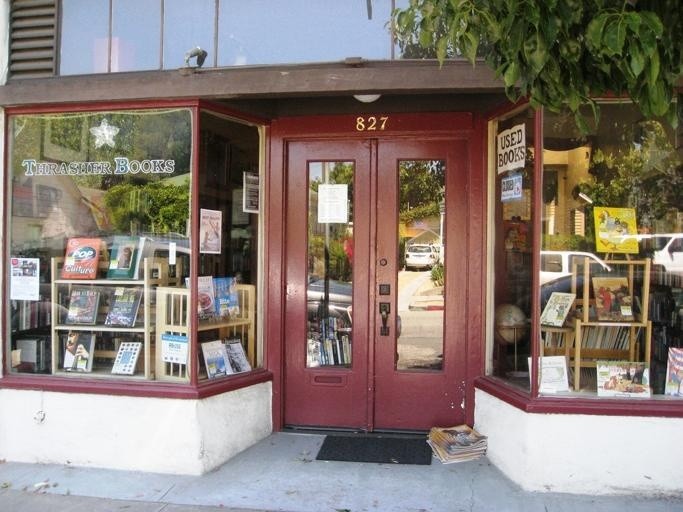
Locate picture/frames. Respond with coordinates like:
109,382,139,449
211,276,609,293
199,208,223,254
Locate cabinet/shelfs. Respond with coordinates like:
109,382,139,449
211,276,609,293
155,284,256,384
50,257,181,381
540,255,652,392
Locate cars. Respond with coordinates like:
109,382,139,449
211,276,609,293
605,230,683,278
541,249,619,290
405,243,440,273
306,262,410,350
30,220,232,291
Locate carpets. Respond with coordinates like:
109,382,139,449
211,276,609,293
313,430,433,467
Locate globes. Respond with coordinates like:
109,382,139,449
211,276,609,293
494,303,531,378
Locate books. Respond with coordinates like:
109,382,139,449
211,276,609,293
528,206,683,397
11,235,252,380
306,317,352,367
426,424,488,464
